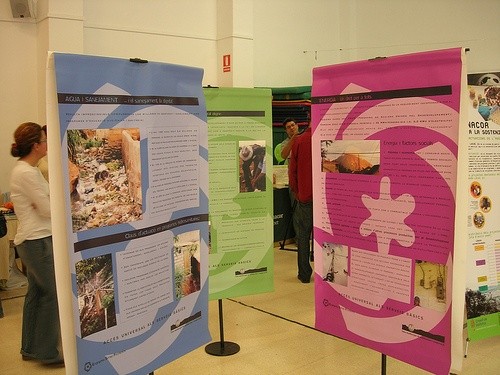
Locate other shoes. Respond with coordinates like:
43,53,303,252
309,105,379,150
21,354,65,368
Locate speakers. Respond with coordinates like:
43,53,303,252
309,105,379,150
10,1,37,18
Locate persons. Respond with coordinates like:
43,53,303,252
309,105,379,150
274,117,302,246
35,124,49,185
288,111,314,283
472,184,490,226
240,144,262,192
9,121,66,369
469,85,500,121
251,148,266,191
